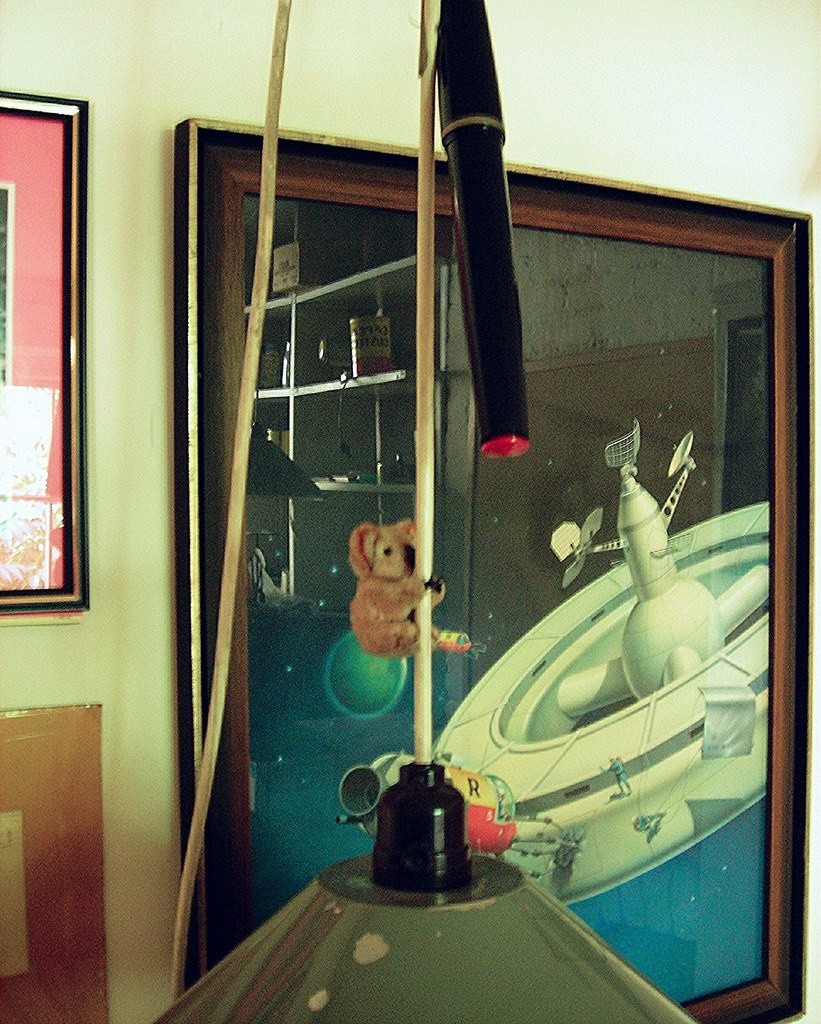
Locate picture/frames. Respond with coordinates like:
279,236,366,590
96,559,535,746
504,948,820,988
0,89,91,614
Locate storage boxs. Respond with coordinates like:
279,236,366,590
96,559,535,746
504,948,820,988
273,240,334,294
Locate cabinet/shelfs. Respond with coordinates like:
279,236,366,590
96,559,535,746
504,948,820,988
239,251,449,622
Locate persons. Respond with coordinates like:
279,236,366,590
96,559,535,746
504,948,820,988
604,755,633,798
631,812,664,835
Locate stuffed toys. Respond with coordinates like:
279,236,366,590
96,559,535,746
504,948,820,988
346,518,447,658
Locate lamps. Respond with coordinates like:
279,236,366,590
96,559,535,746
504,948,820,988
152,0,705,1024
